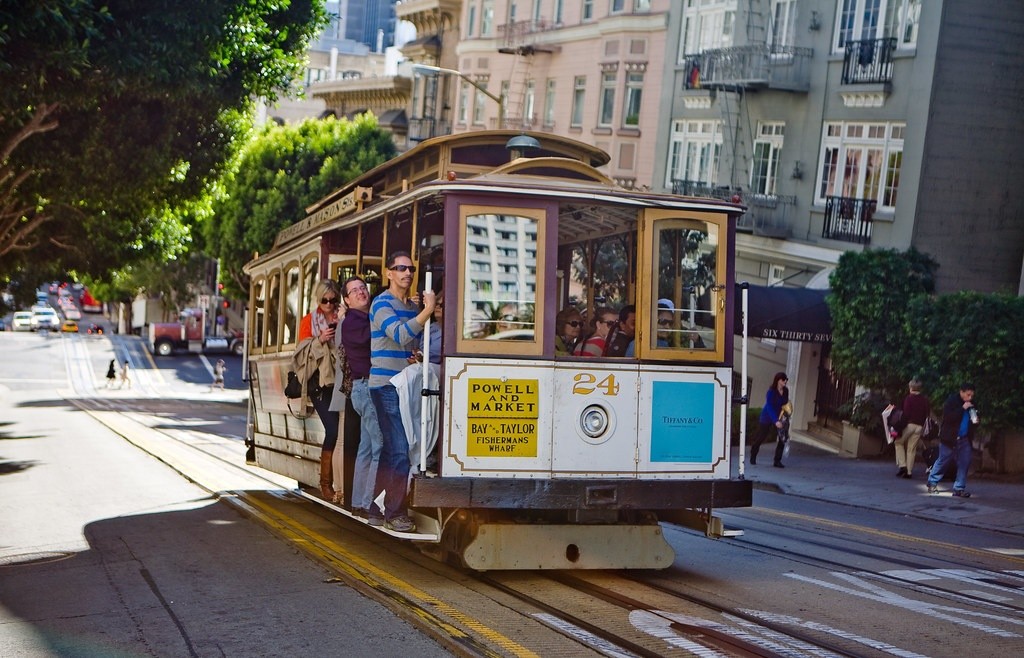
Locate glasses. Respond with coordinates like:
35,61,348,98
567,321,584,327
658,319,674,327
494,311,519,323
389,264,416,273
602,321,615,328
320,297,339,304
780,378,789,382
346,286,365,297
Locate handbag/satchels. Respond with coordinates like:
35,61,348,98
283,371,322,420
923,418,940,441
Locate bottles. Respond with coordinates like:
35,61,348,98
969,407,978,424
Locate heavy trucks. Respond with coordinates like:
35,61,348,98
147,295,244,356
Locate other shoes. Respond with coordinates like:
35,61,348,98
773,461,786,468
927,482,939,494
352,507,369,519
895,467,913,479
952,489,970,498
750,456,757,464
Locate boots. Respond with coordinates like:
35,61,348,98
320,451,336,501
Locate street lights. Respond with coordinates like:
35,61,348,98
410,63,504,130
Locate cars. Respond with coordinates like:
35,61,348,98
12,282,104,335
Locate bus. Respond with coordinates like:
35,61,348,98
241,129,756,574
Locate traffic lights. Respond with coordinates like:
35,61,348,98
223,300,230,308
219,283,224,291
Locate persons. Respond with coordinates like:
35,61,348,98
927,383,981,497
104,359,132,391
891,379,930,478
750,372,791,468
209,360,227,391
470,298,706,358
294,252,446,532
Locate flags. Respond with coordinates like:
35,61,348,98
688,56,703,89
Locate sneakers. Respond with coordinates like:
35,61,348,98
368,516,384,525
383,515,417,532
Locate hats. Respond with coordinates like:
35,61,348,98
658,298,675,313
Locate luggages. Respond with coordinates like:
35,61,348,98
924,447,958,482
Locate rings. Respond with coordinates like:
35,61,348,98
691,333,693,337
